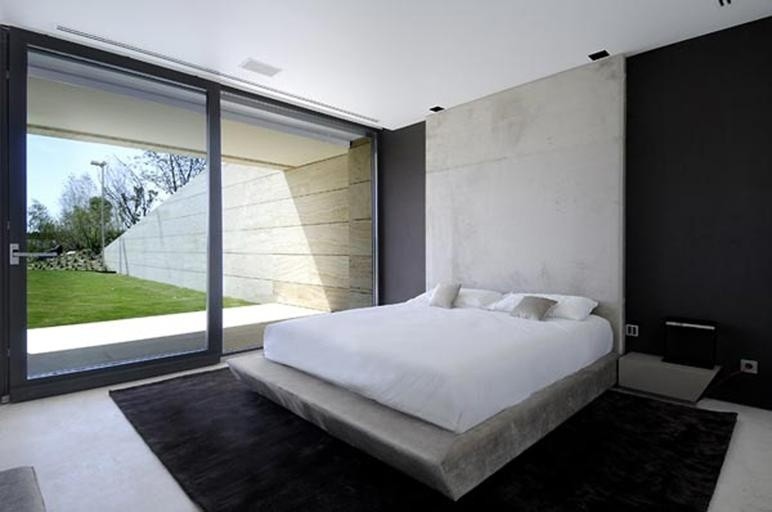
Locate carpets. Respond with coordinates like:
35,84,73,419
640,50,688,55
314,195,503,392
110,365,737,511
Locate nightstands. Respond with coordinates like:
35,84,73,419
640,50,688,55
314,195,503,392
618,350,722,404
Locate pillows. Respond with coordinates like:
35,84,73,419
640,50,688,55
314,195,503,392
414,282,599,322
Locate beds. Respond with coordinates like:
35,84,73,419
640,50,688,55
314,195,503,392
227,300,617,502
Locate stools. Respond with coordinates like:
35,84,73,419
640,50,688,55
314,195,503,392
0,466,47,512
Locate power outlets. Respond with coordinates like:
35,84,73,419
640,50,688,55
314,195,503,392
741,359,758,374
